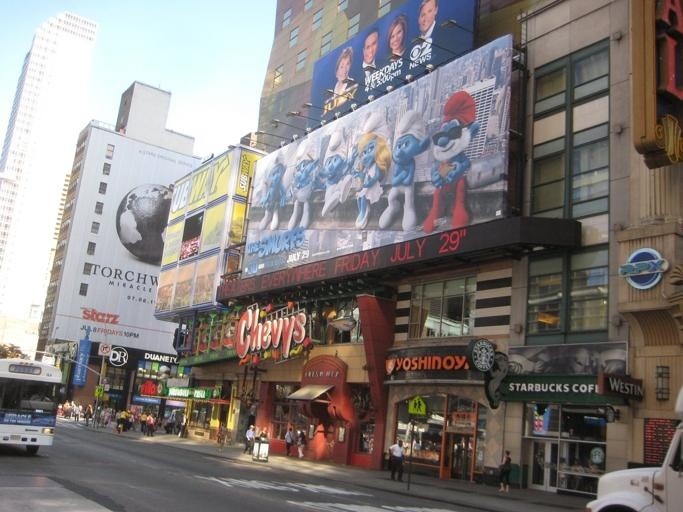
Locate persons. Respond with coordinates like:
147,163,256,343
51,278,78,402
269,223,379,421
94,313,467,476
384,12,407,60
295,429,305,458
412,438,420,456
558,456,599,492
56,400,188,438
497,449,512,493
28,385,51,402
387,439,404,482
354,27,378,82
242,424,255,455
284,426,295,458
258,426,267,442
414,1,439,42
216,423,226,446
319,46,354,108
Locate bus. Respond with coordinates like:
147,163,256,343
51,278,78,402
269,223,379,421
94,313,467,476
0,358,63,457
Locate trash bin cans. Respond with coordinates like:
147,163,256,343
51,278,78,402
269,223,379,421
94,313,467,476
252,440,270,462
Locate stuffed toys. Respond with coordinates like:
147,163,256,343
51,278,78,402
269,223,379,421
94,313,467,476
255,91,481,237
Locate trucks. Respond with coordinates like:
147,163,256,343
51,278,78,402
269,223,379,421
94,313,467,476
585,386,682,512
